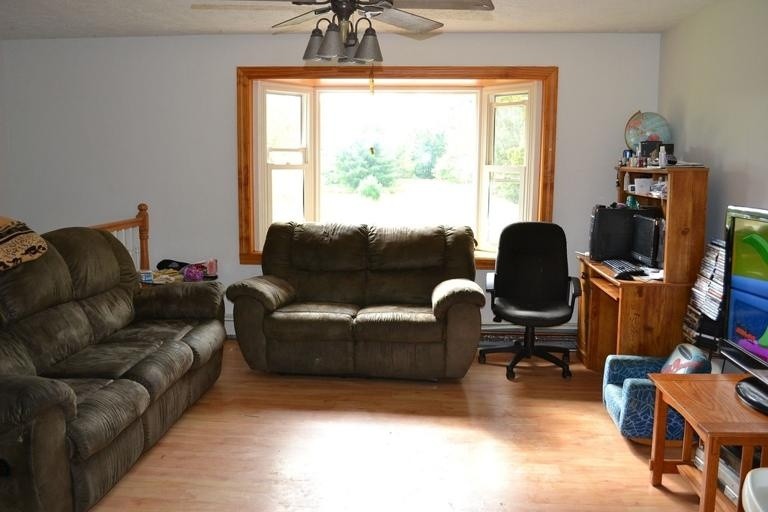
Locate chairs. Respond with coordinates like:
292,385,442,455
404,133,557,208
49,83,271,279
602,342,712,449
478,222,582,380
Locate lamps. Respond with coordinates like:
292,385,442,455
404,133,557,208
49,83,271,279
302,13,383,64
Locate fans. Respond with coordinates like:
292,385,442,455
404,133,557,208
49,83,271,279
272,0,495,34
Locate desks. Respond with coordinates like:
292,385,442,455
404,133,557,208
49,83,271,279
646,373,768,512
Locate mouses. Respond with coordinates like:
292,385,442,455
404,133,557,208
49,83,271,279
615,272,634,280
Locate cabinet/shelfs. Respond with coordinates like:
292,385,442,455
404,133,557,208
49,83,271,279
577,164,708,375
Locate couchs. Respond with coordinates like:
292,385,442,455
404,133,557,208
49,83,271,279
226,221,486,383
0,226,227,512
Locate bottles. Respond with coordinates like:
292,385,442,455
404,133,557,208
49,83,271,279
658,145,667,167
622,172,631,191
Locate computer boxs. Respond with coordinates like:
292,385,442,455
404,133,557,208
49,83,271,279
589,205,665,265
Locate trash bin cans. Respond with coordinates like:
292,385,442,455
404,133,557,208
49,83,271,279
741,467,768,512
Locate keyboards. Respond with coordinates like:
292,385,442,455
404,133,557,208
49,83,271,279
602,259,644,274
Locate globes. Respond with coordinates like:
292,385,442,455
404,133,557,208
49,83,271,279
624,110,673,154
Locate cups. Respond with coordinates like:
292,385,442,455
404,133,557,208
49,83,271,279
628,177,651,195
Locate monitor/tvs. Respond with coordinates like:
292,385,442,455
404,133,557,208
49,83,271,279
719,204,768,417
630,214,661,268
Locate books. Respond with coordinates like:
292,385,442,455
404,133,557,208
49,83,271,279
682,238,726,343
